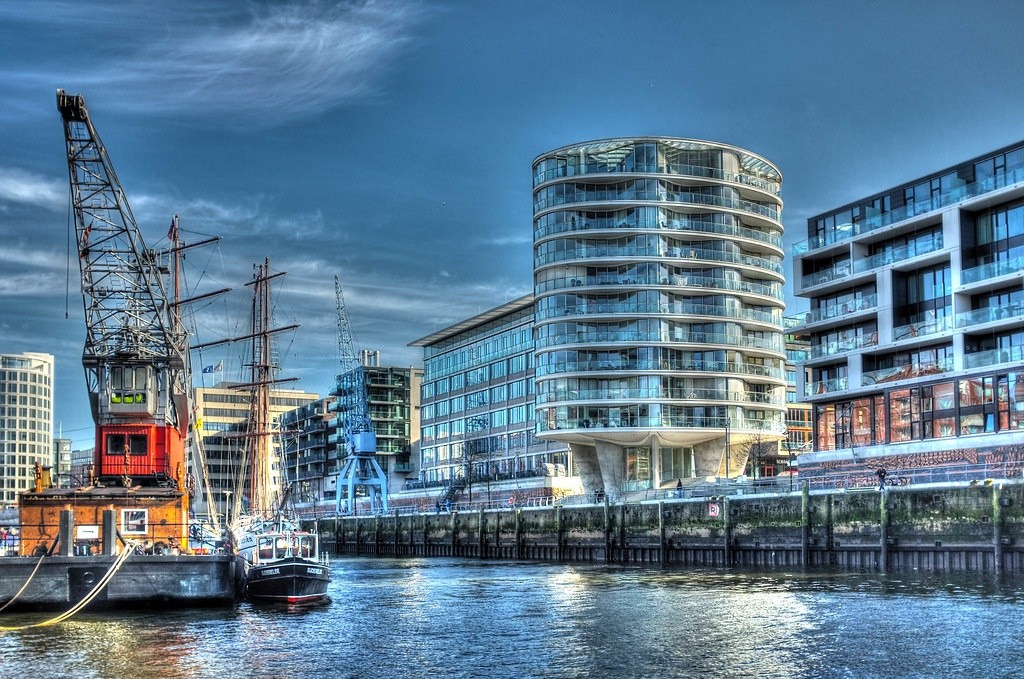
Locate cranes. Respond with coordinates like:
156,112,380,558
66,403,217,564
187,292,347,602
329,272,389,517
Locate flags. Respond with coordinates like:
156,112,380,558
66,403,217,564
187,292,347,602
203,360,223,375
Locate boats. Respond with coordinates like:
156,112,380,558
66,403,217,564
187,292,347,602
0,83,242,611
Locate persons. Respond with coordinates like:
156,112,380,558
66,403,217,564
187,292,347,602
446,498,451,514
435,499,440,514
676,478,683,498
0,527,7,549
593,488,605,505
872,466,887,491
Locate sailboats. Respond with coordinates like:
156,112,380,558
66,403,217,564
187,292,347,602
216,256,334,604
